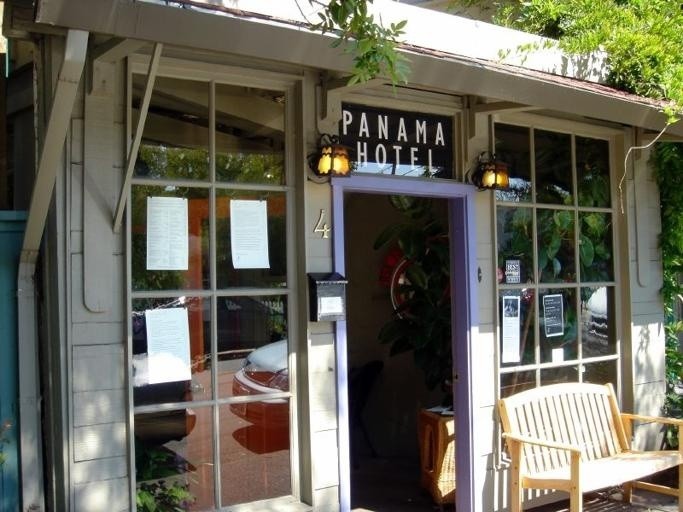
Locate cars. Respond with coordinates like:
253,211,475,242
230,339,289,428
582,286,610,356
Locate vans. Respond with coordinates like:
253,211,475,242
134,380,196,444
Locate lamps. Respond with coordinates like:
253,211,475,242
308,131,352,185
471,150,507,191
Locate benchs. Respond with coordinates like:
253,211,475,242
495,381,683,512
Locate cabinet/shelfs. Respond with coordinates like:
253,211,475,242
417,408,456,512
